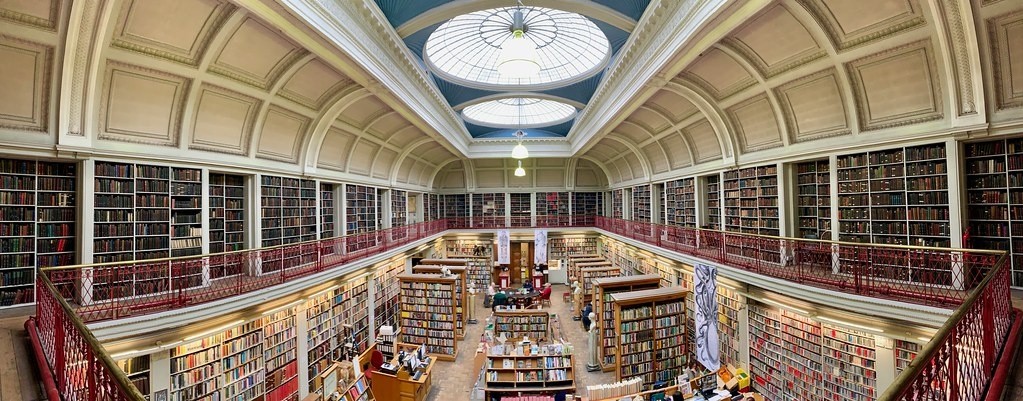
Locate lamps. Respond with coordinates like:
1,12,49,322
514,161,526,176
511,98,529,159
497,1,542,79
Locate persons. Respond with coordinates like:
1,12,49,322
489,282,495,293
537,283,551,302
522,280,533,291
573,281,581,293
688,362,704,379
587,313,599,367
493,287,506,304
582,301,592,331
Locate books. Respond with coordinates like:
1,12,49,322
400,272,463,355
373,260,405,338
67,354,150,401
306,276,370,401
0,157,405,306
423,192,603,231
685,276,948,401
613,135,1023,288
549,238,687,401
447,240,493,293
477,315,573,401
170,306,298,401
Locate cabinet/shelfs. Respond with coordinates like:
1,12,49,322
0,131,1023,401
